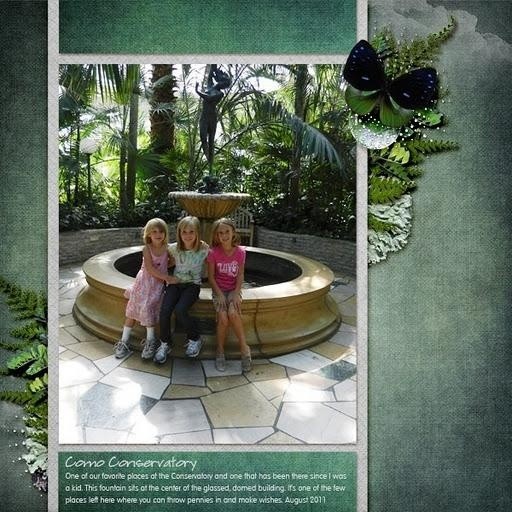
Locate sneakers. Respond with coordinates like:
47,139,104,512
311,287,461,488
115,339,131,359
141,339,161,360
154,342,172,364
185,335,206,358
240,345,253,372
215,351,226,372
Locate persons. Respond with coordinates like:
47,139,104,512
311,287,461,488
112,217,210,358
195,64,232,177
153,216,213,364
204,217,252,372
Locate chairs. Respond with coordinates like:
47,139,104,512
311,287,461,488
222,207,255,247
